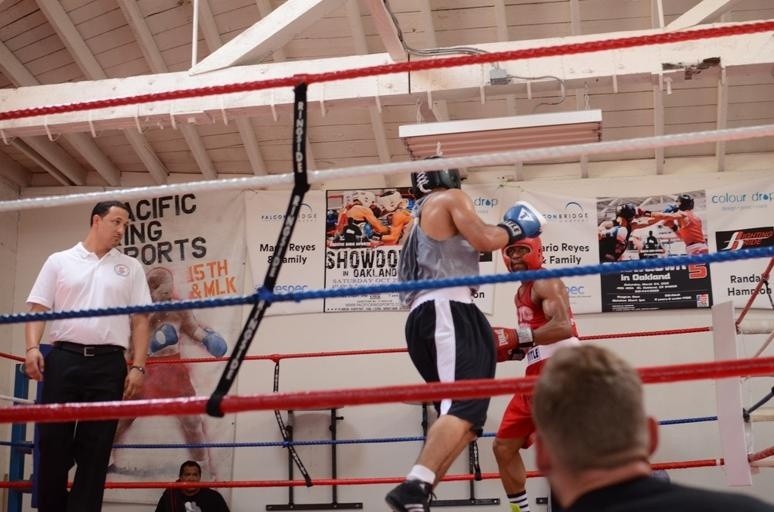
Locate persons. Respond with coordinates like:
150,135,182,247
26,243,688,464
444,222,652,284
154,460,231,512
22,201,159,511
598,202,678,263
385,155,546,511
370,191,413,247
487,237,584,511
631,194,709,256
328,190,391,242
104,266,228,482
529,343,774,510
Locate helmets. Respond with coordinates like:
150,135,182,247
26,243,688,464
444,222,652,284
676,194,694,211
352,189,375,208
615,202,635,223
377,191,402,212
411,156,461,200
501,235,544,273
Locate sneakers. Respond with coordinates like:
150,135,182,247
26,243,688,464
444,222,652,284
385,479,437,512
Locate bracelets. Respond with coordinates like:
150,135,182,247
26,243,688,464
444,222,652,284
129,365,146,374
26,346,40,352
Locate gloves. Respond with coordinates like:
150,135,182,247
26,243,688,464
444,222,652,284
491,327,535,363
151,323,178,352
633,207,651,217
600,226,628,242
663,203,678,213
200,326,227,357
497,201,547,246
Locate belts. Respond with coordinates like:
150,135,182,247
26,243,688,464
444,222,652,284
52,341,123,357
